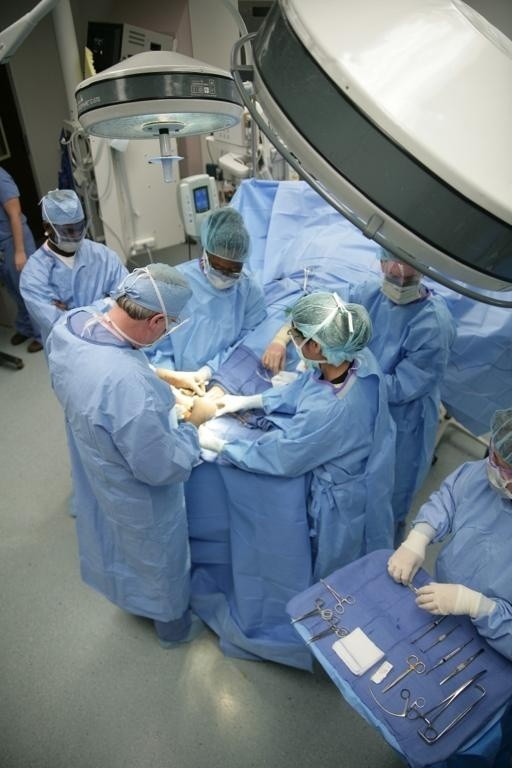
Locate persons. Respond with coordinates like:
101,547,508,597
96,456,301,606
45,263,217,646
388,406,511,660
198,293,397,581
349,246,456,548
19,190,130,336
1,164,44,353
141,207,266,380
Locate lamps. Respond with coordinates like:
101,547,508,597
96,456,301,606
232,0,511,307
76,50,242,153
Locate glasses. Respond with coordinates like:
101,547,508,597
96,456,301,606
209,260,241,278
489,448,512,477
287,329,302,341
61,223,84,234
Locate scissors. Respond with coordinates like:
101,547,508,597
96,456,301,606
291,578,356,646
382,654,426,693
406,669,487,741
367,686,426,718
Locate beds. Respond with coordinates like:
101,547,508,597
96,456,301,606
149,181,450,568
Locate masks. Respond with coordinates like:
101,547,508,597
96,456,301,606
487,461,512,501
49,225,85,253
203,250,245,290
381,280,421,305
291,336,328,367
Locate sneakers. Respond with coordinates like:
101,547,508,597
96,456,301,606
156,613,205,648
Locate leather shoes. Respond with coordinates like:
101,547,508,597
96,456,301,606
11,332,43,352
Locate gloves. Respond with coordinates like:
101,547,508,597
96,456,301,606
199,394,262,452
271,371,298,387
387,523,436,586
415,582,496,618
